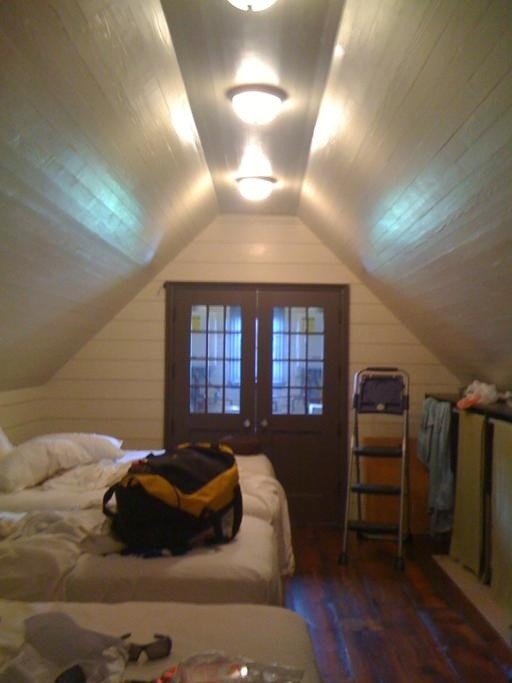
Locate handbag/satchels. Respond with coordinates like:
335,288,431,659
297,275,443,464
102,440,248,560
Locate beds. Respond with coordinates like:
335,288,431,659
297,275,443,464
1,430,309,682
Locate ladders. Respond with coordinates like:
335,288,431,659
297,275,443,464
340,366,412,568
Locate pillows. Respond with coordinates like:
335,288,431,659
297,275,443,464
0,430,125,494
